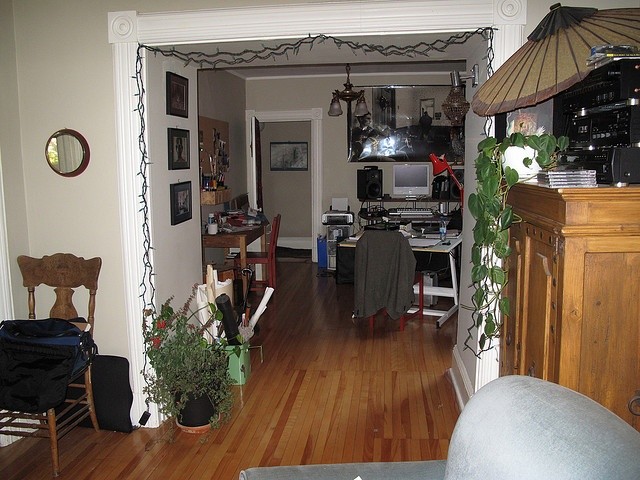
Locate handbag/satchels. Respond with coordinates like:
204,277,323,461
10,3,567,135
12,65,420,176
0,317,97,414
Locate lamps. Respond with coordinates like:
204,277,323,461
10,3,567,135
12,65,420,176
328,63,369,117
442,64,479,127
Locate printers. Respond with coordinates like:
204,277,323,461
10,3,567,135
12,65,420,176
321,197,354,225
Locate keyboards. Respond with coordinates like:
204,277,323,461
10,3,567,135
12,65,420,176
389,208,436,219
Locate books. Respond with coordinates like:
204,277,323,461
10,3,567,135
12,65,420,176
537,169,598,189
344,231,365,243
407,238,442,247
422,229,462,239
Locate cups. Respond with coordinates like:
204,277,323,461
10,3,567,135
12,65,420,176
207,223,218,235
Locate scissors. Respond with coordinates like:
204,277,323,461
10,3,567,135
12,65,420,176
442,240,451,245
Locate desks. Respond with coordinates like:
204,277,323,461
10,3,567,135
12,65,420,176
202,218,269,303
339,231,463,329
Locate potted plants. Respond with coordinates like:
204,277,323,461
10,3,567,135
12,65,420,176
140,284,251,435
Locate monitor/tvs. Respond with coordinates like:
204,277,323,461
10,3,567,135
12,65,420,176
390,162,434,201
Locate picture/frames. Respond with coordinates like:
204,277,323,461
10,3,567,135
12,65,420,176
269,141,308,172
169,180,192,227
167,127,191,170
165,70,189,119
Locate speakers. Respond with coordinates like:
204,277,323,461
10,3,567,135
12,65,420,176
356,166,384,201
447,164,464,202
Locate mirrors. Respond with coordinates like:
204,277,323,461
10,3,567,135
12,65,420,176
45,129,90,178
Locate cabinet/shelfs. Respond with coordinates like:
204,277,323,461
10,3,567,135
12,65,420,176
498,179,640,431
362,197,458,232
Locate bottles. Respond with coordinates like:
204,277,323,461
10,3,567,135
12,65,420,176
208,213,214,224
440,218,446,242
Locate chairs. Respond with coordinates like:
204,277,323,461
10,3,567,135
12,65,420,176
234,214,281,294
1,253,102,480
368,272,424,338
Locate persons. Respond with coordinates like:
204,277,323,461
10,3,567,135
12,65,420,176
419,111,433,136
352,111,385,161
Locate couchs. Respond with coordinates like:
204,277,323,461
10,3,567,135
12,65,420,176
238,375,640,480
